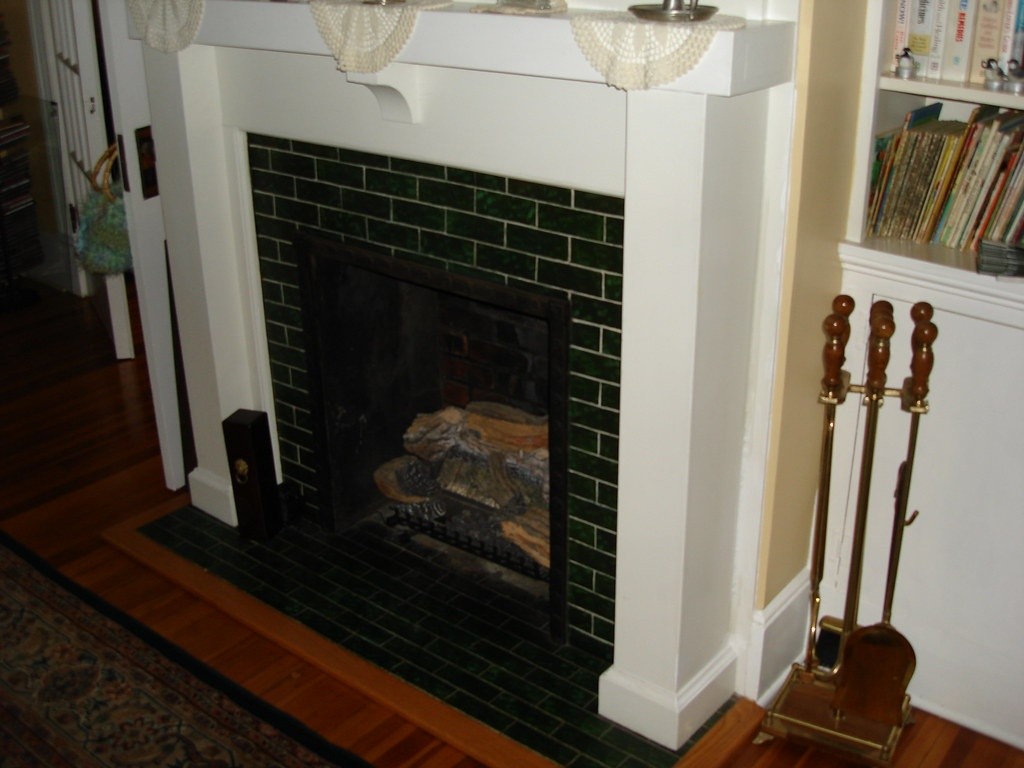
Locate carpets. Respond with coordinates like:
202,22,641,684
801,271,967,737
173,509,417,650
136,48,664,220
0,528,375,768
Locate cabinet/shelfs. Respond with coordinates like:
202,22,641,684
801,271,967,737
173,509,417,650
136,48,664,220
835,0,1024,331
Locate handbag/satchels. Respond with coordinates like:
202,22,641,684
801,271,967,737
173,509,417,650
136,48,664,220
75,146,131,274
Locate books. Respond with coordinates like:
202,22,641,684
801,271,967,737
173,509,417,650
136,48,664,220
891,0,1024,85
0,11,44,276
865,103,1024,251
